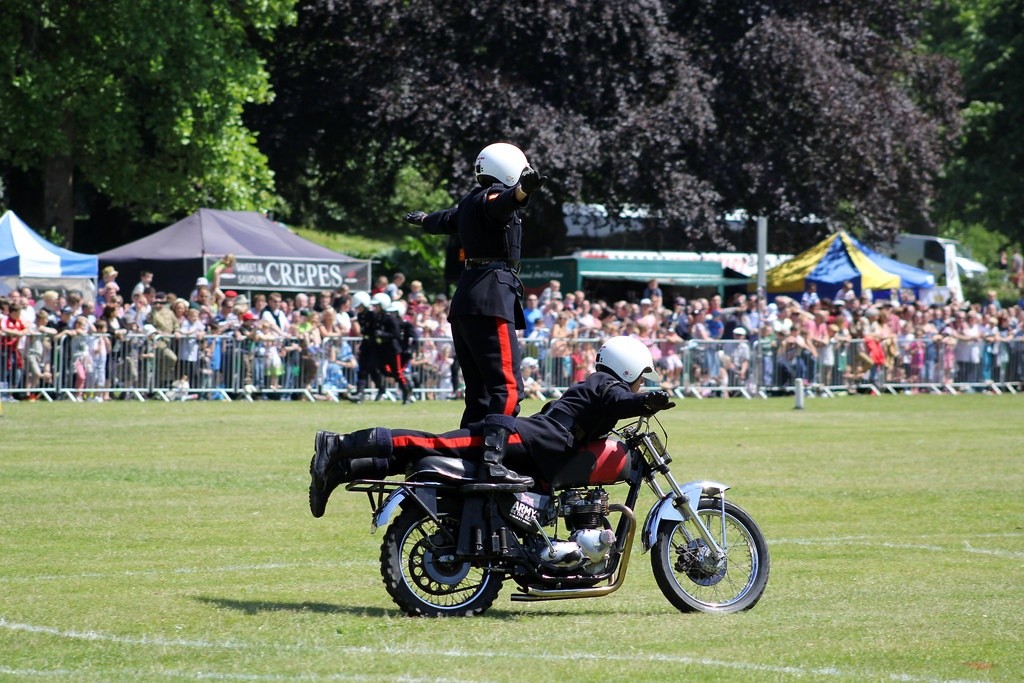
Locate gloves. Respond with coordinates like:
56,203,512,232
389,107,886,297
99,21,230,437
519,167,547,193
644,390,675,410
402,211,428,226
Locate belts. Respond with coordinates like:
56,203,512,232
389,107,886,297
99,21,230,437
463,258,521,273
543,407,592,446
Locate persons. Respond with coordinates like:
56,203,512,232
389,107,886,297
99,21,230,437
1010,248,1024,288
309,336,677,518
517,280,1024,401
998,250,1009,283
406,143,547,487
0,266,466,404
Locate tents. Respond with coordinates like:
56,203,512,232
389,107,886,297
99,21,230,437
748,230,935,310
98,209,372,303
0,210,99,306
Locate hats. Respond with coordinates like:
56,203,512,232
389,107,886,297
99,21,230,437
242,313,256,321
197,277,208,286
103,266,118,278
226,291,238,298
733,327,746,335
61,306,73,314
172,298,190,308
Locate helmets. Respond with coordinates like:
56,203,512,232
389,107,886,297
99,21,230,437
595,335,659,384
351,291,371,310
371,293,391,311
387,301,407,318
474,143,531,188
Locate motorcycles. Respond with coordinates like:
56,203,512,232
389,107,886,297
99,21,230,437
343,387,773,619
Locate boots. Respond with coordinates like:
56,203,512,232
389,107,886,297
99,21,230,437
310,427,392,496
480,414,534,485
309,456,389,519
